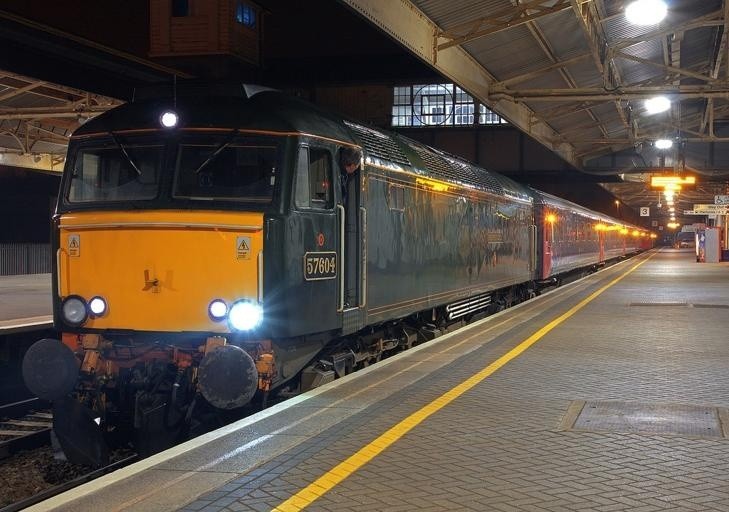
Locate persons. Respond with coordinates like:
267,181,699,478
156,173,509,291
338,144,362,207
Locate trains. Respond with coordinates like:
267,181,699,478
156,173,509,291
21,79,659,465
